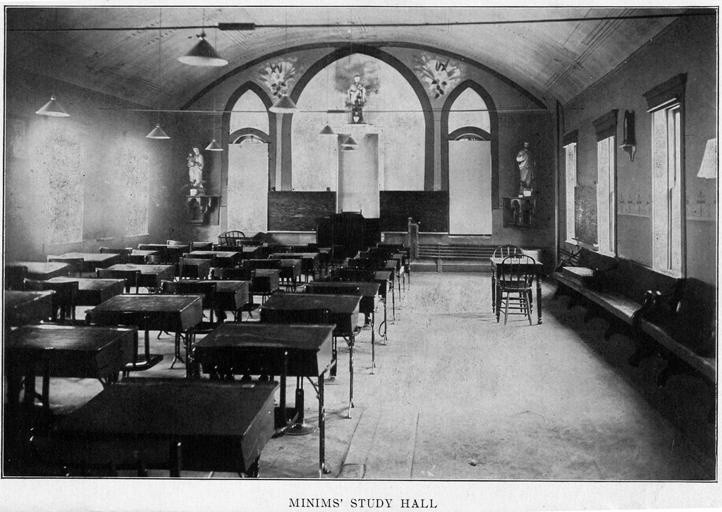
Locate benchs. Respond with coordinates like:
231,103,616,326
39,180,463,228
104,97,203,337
546,244,717,422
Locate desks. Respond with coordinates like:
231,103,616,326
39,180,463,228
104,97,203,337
488,256,545,325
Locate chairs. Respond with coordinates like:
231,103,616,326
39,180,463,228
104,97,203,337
499,253,533,326
492,243,533,313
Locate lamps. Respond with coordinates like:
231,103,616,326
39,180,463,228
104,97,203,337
694,137,718,180
320,8,359,153
141,9,176,141
33,95,72,118
269,12,301,114
202,75,225,153
176,7,231,69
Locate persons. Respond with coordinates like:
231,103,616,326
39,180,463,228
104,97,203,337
345,75,367,124
187,145,205,193
515,142,536,195
